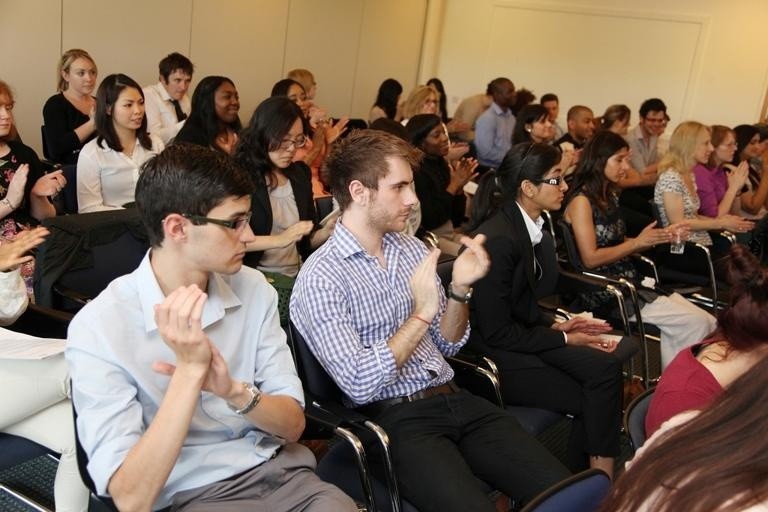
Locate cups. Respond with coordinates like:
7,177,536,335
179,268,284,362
669,228,687,254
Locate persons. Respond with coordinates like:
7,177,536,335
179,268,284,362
66,68,767,511
0,48,245,290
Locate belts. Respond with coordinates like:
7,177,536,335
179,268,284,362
369,381,460,412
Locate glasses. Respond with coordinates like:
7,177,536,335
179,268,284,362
537,176,565,185
282,136,307,149
646,118,663,123
181,211,252,231
